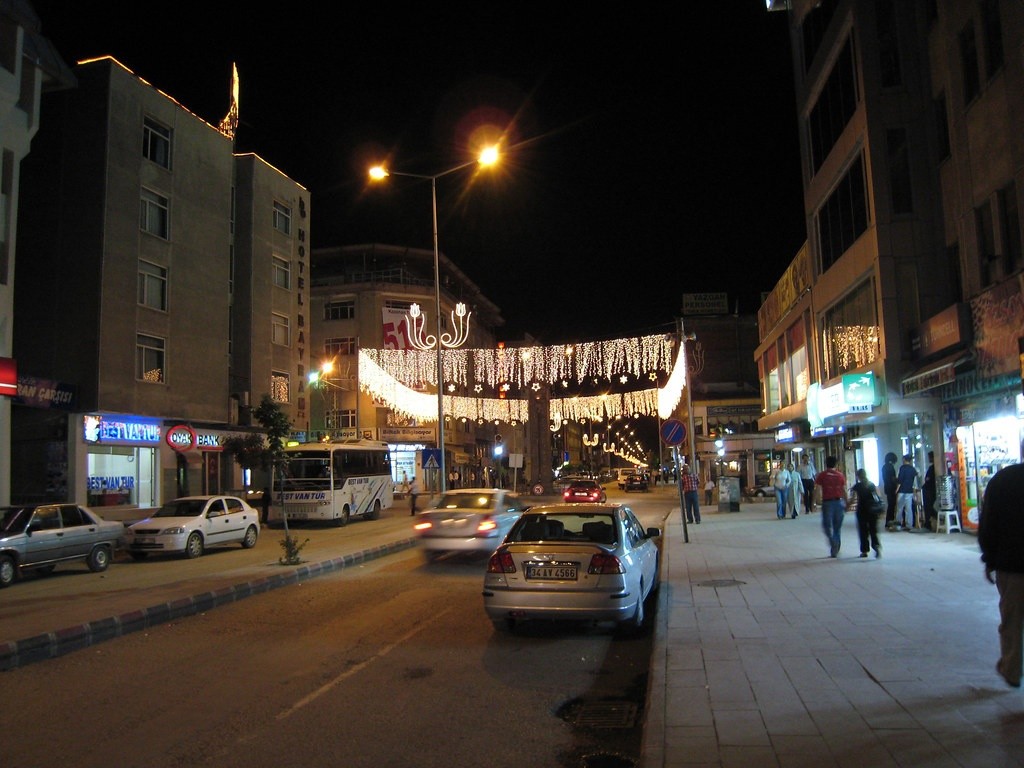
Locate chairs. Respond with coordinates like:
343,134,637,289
583,521,605,539
539,520,565,537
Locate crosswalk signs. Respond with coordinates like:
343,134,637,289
421,449,441,468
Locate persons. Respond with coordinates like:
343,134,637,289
400,471,409,492
702,476,715,506
449,470,458,490
480,471,486,488
882,451,938,531
847,469,882,559
976,437,1024,689
811,456,849,558
470,471,475,488
681,464,701,524
407,477,420,516
769,455,817,520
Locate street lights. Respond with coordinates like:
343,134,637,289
584,393,650,469
715,439,726,475
367,138,507,487
307,362,337,439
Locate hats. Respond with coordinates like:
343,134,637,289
682,464,688,469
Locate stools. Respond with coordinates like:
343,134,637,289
936,510,962,535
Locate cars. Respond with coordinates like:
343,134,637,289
482,503,662,636
564,480,607,504
412,489,530,564
0,502,124,586
575,470,613,480
625,474,648,493
750,483,777,498
122,495,261,560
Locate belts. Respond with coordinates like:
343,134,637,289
824,497,846,501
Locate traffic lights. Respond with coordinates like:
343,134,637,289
494,434,503,458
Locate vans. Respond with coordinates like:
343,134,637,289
617,468,637,490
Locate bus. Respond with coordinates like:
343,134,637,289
266,439,396,526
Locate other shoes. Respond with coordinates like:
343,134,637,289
791,515,795,518
804,511,809,514
888,521,903,526
903,528,913,532
875,549,883,559
686,520,694,524
859,552,868,557
778,516,786,520
810,507,813,512
995,659,1022,688
696,521,700,524
831,540,841,558
